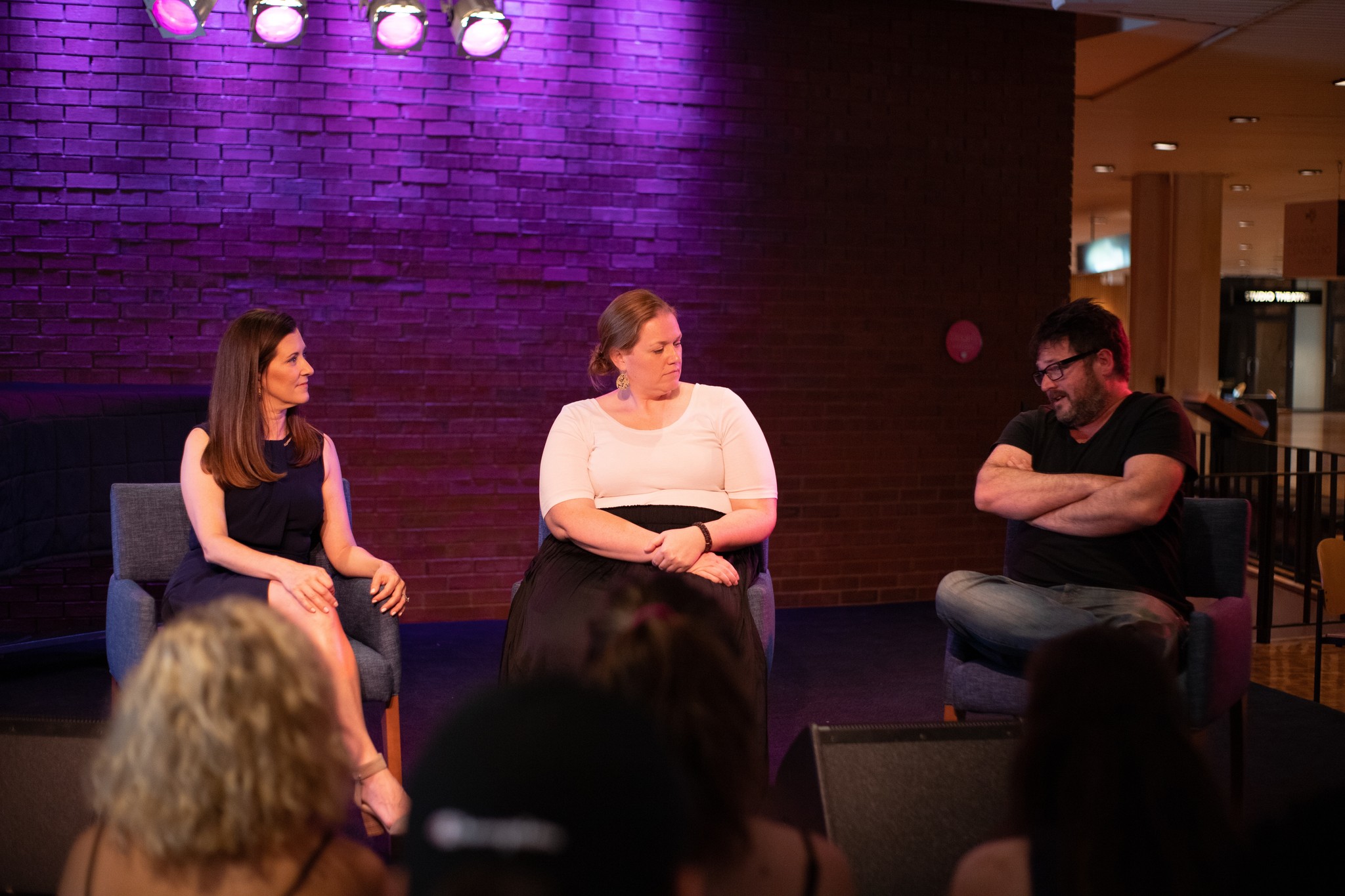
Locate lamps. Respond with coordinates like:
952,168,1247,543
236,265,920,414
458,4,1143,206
144,0,217,40
239,0,309,49
359,0,429,56
440,0,514,62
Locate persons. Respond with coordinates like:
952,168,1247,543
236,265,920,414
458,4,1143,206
58,594,391,895
384,606,848,895
948,620,1237,896
936,296,1200,681
162,308,411,834
498,291,780,683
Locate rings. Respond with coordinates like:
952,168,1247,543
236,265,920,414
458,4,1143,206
401,594,410,602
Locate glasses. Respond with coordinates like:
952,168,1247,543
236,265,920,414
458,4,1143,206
1031,350,1096,387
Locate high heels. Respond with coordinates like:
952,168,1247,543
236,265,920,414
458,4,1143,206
353,752,414,840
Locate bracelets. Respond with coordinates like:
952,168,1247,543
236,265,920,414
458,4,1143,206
692,520,713,553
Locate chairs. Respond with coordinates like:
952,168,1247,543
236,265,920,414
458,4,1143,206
107,484,401,787
539,512,776,687
1314,536,1345,705
941,500,1250,730
